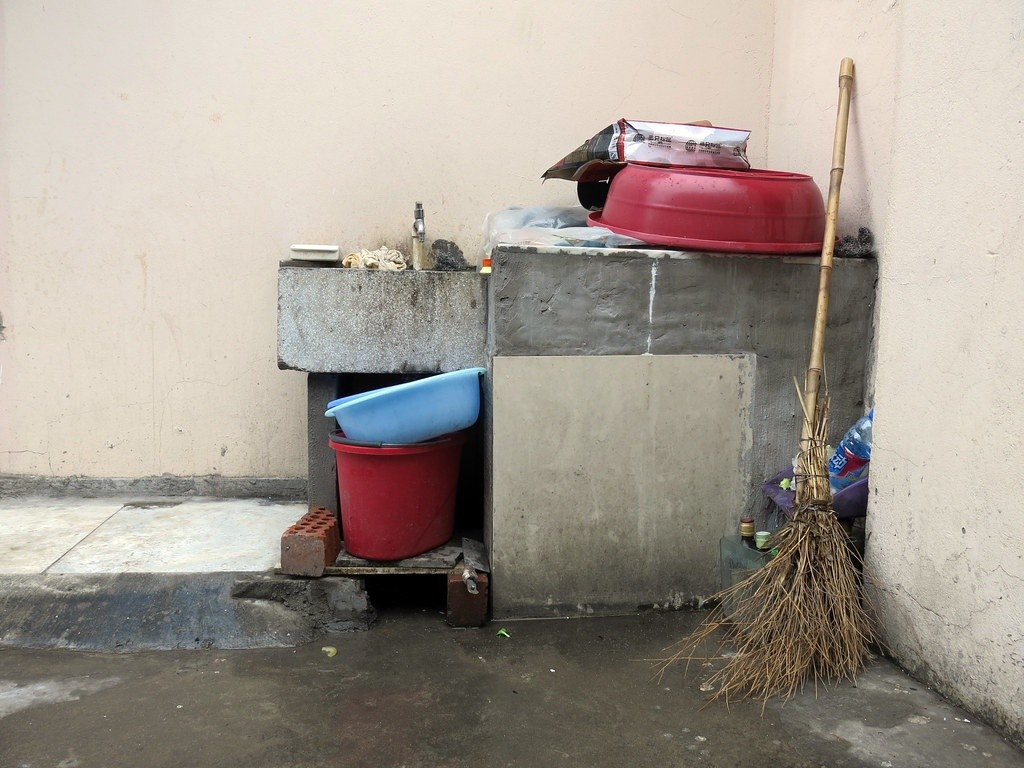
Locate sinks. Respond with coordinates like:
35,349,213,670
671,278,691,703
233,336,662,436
277,264,487,370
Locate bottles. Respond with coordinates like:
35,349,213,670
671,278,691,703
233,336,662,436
478,258,491,273
737,517,778,557
830,409,873,494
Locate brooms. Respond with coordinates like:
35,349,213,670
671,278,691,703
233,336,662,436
647,56,897,708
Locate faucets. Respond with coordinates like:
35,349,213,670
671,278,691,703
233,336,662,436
410,200,426,242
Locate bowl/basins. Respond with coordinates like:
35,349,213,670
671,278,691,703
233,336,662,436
324,367,486,445
586,163,840,253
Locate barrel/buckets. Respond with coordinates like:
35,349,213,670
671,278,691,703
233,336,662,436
328,432,464,559
